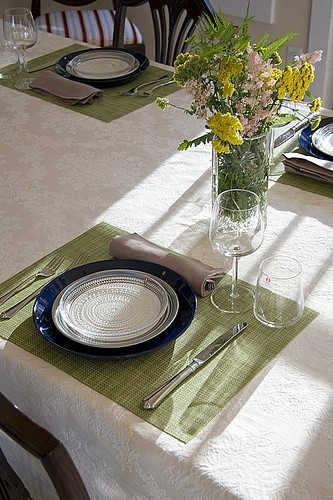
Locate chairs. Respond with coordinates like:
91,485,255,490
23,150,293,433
31,0,146,56
113,0,219,72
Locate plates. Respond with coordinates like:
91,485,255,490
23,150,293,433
32,258,197,362
52,269,179,348
66,50,139,79
54,45,149,85
298,114,333,161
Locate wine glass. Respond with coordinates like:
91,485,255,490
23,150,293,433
3,7,38,91
209,189,267,315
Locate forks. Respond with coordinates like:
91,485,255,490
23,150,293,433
116,75,169,97
0,253,64,307
0,251,89,321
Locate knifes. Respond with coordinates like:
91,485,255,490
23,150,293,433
139,320,250,410
270,107,321,148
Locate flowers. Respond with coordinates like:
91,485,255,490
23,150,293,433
156,0,322,218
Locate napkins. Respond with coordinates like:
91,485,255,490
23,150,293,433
109,233,227,296
28,71,103,106
282,153,333,183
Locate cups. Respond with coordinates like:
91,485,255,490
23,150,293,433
253,254,305,328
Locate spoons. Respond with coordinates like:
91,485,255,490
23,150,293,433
135,77,178,96
0,57,59,80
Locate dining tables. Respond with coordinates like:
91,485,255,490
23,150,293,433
0,18,333,500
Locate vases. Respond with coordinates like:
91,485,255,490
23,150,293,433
208,128,272,255
